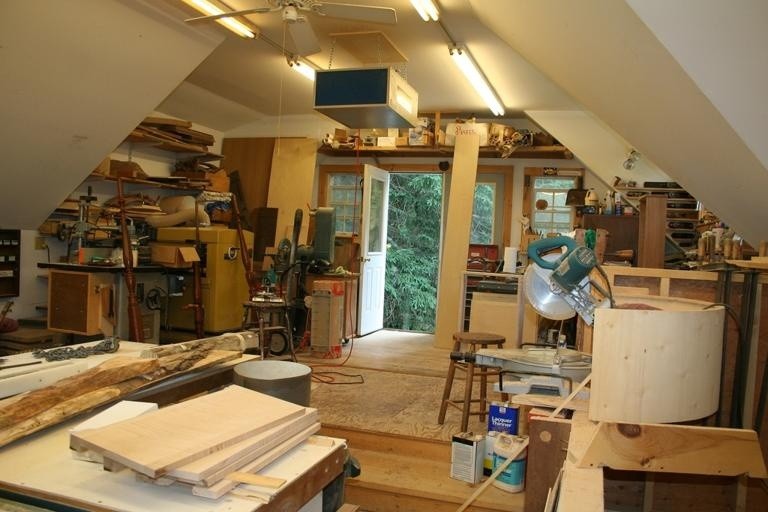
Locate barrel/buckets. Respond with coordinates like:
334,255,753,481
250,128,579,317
486,431,527,492
234,360,312,406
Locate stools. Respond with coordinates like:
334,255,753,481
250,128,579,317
439,333,508,433
242,301,296,361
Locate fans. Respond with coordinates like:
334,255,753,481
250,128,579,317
183,0,398,59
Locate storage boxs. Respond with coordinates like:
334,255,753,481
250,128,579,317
152,243,201,268
445,123,491,147
409,117,435,147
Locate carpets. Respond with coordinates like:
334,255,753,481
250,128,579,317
301,359,510,445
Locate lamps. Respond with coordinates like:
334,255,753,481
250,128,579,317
410,0,442,23
447,41,505,117
624,150,640,170
522,236,614,325
286,54,324,81
183,0,260,41
283,4,298,24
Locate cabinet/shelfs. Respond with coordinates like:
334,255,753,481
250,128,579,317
51,132,225,216
